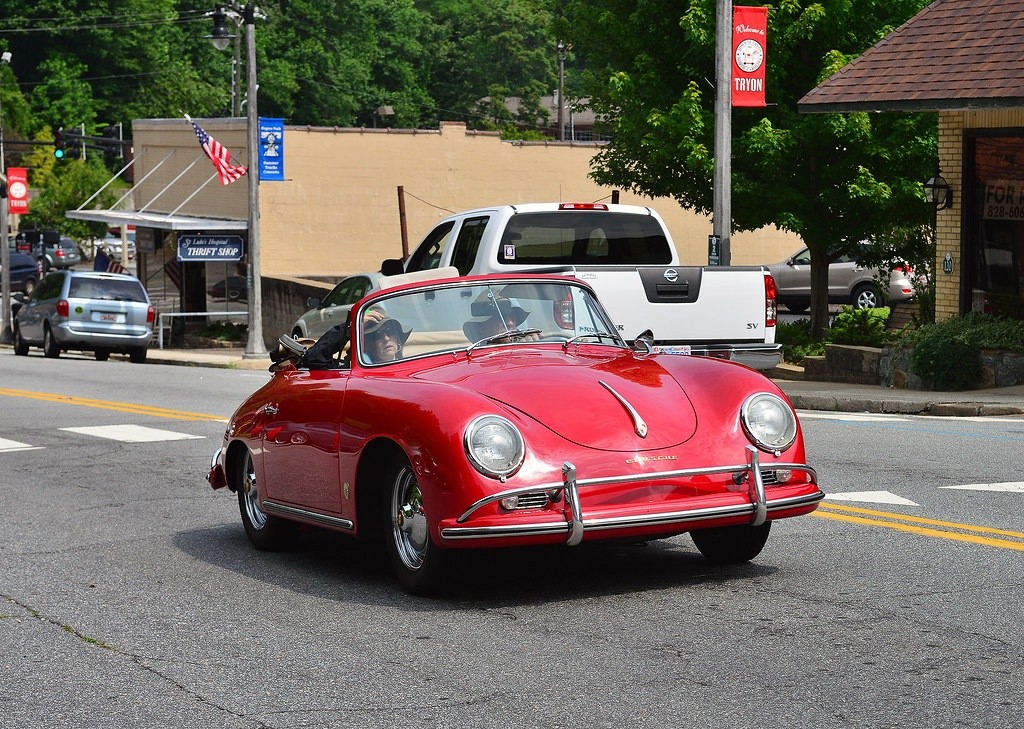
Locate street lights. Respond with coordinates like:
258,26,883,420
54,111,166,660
205,1,272,360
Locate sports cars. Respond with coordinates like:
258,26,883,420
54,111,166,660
206,273,826,601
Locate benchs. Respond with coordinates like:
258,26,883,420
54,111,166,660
331,329,474,361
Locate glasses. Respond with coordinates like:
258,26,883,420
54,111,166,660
369,326,393,341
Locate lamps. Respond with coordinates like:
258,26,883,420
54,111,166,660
922,156,953,211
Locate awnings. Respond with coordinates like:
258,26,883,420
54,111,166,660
65,209,248,232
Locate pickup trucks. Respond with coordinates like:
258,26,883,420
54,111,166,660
290,202,785,375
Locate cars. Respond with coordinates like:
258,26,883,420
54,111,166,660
765,235,932,317
0,251,41,294
83,229,136,264
9,268,156,363
7,234,81,273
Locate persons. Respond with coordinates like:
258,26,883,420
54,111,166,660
463,295,589,345
302,303,413,368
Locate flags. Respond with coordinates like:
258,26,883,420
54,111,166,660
185,114,248,186
93,247,124,275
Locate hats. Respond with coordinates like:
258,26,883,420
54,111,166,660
362,305,413,355
463,286,531,343
